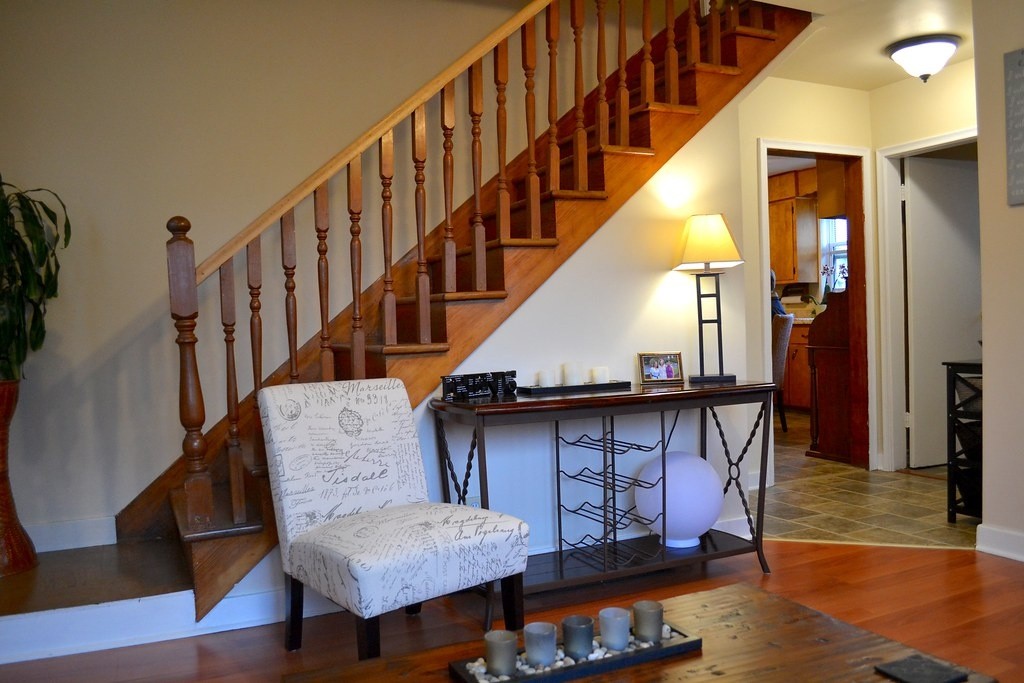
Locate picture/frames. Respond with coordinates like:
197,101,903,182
638,350,686,386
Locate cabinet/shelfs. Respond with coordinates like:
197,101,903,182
782,322,813,408
815,159,846,220
941,360,983,523
767,167,819,286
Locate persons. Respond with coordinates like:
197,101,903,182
658,357,667,379
666,360,674,378
771,268,788,320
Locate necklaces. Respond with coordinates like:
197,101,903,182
649,360,660,379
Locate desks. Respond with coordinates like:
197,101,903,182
426,372,780,632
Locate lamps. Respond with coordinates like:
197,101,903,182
634,451,726,548
678,211,750,382
883,31,963,84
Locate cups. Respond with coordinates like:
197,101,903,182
523,622,558,669
484,629,518,677
599,607,631,651
561,615,594,660
631,599,664,644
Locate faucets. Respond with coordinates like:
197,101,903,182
799,293,821,307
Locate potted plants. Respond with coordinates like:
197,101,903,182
0,175,77,577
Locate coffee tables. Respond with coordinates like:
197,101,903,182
274,581,1001,683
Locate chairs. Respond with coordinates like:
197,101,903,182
771,313,794,433
256,374,530,662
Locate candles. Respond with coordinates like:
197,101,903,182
538,370,555,388
562,362,584,386
592,366,608,383
